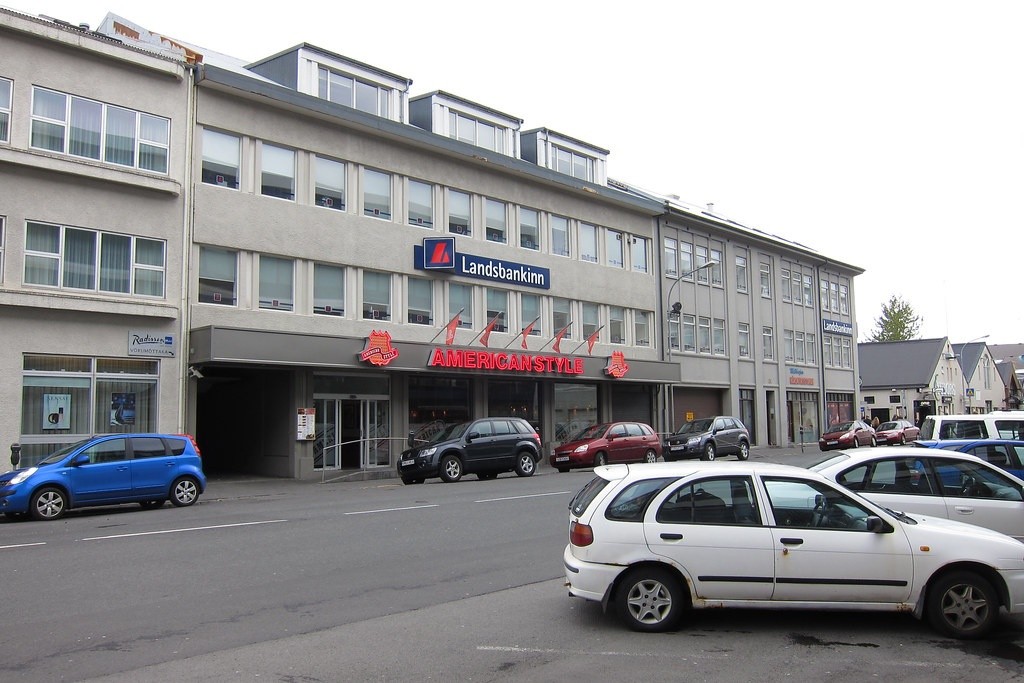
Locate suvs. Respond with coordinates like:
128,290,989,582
662,416,751,462
397,416,544,486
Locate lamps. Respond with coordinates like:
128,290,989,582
670,302,683,315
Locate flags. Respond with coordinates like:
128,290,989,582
479,319,497,347
552,328,567,353
588,331,598,355
521,321,535,349
446,315,460,345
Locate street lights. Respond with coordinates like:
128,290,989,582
960,335,990,413
667,260,716,434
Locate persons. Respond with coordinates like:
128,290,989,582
872,417,879,430
892,415,897,421
864,415,872,427
915,419,919,427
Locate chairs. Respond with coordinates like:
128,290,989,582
964,424,980,439
892,465,913,490
992,452,1007,465
919,470,942,495
731,485,752,521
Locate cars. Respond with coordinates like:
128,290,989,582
819,420,877,451
743,446,1024,544
549,422,662,474
564,461,1024,641
0,433,207,521
875,420,921,446
911,438,1024,491
114,404,135,424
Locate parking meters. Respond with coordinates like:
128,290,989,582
10,443,22,471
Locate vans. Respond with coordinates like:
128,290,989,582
921,410,1024,467
